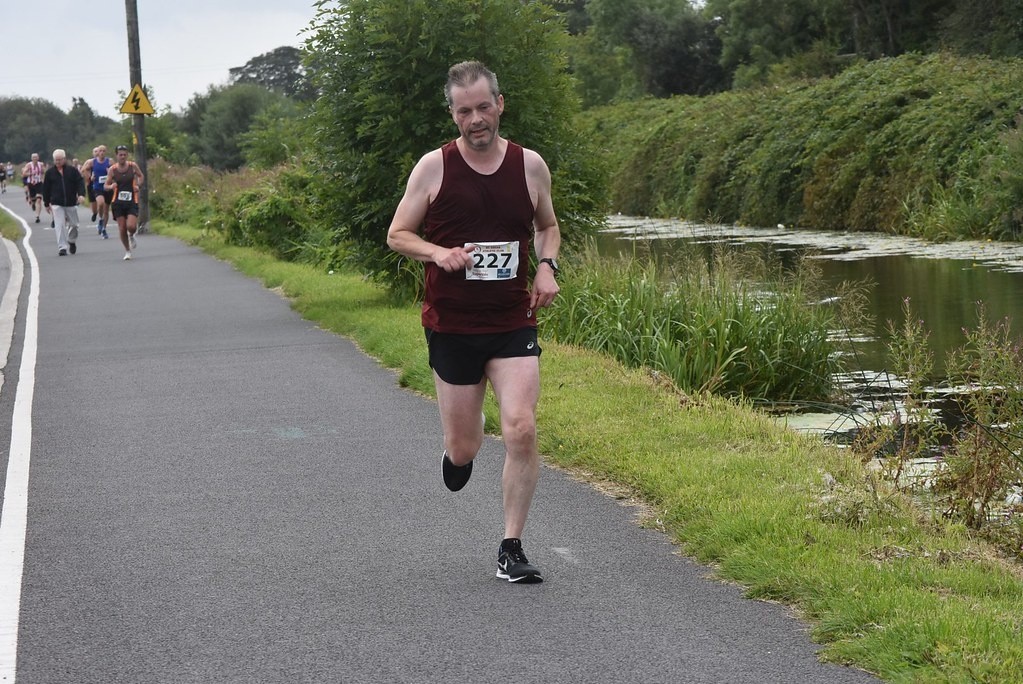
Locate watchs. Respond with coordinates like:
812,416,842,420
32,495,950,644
540,258,558,270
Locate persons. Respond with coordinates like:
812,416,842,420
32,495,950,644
81,147,98,222
85,144,115,239
0,163,6,193
71,158,83,171
42,149,86,256
387,62,561,582
23,153,44,223
21,161,31,204
7,162,14,179
103,145,145,260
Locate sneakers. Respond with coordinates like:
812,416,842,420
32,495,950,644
495,539,543,583
441,412,485,493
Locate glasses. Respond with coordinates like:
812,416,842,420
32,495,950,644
117,145,127,150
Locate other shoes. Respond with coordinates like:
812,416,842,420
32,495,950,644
51,221,55,228
92,214,97,221
68,241,76,254
124,253,132,260
98,220,104,231
128,232,136,249
35,219,40,223
32,204,35,211
59,249,67,256
101,229,108,239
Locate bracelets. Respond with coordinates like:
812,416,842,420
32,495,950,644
137,184,141,189
